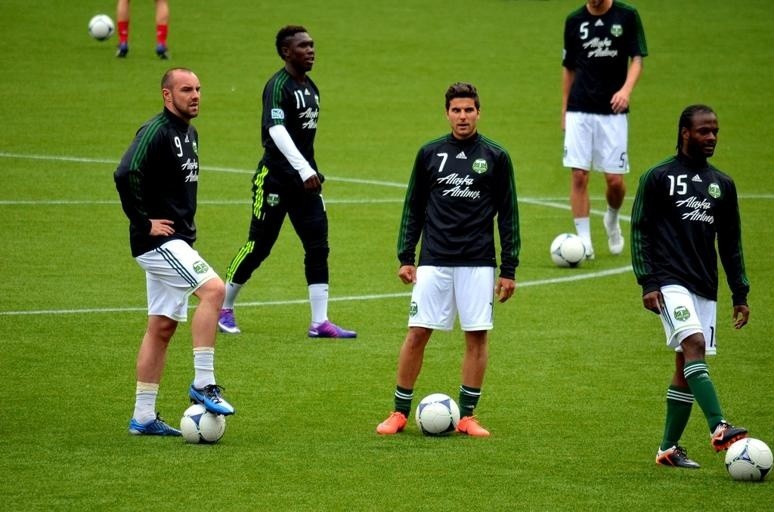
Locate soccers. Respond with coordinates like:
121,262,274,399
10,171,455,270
416,393,460,435
726,437,770,482
89,15,114,41
550,234,587,270
180,404,224,441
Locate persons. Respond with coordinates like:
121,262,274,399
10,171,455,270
374,82,522,440
215,23,358,343
111,67,238,437
629,104,753,470
559,1,651,262
114,1,170,60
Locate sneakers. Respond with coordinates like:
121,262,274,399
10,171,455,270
709,419,749,453
455,414,490,437
218,307,238,334
655,444,701,470
127,415,183,437
576,230,595,258
115,43,128,57
375,410,408,436
188,382,236,417
156,44,168,60
307,318,358,339
603,213,625,256
